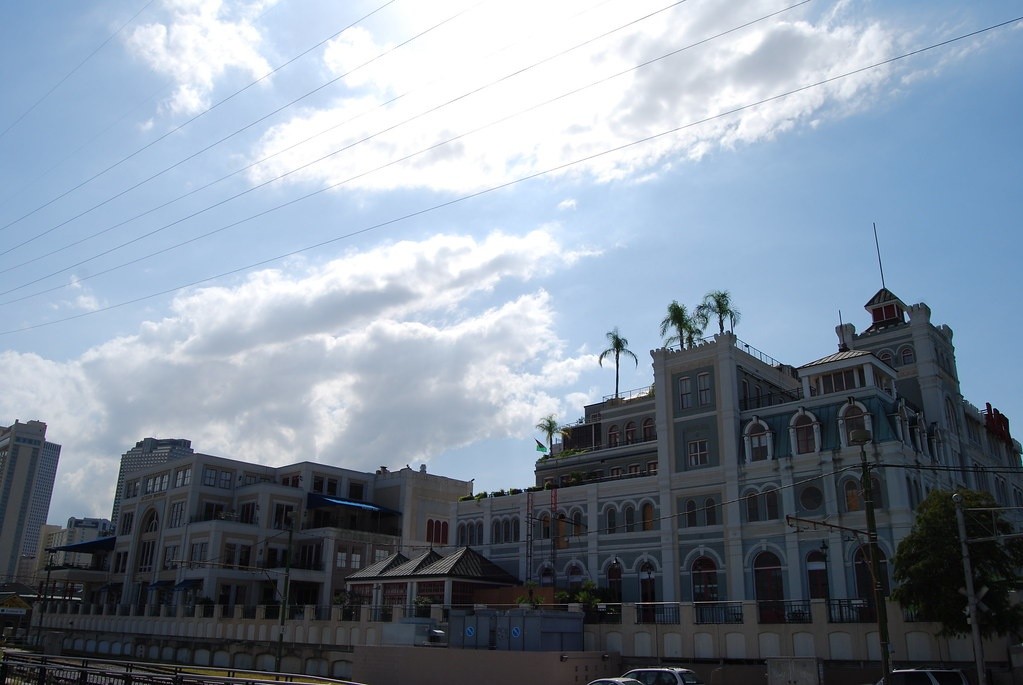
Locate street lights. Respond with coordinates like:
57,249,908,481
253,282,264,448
850,429,891,678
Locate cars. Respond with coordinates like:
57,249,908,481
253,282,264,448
587,678,644,685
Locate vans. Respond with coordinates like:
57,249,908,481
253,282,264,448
621,667,702,685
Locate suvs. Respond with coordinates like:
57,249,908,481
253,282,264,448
874,664,968,685
